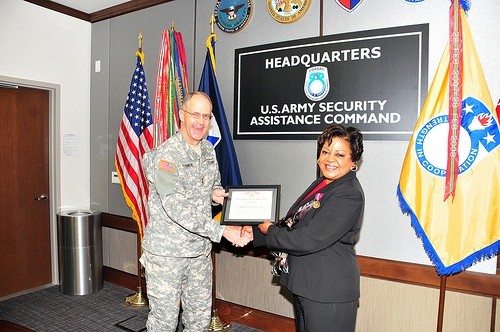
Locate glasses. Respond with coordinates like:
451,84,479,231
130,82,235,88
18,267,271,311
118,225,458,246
180,107,212,120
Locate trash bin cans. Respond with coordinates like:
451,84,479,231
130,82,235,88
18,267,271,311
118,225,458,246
56,209,106,296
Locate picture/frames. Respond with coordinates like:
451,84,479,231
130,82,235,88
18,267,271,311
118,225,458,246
221,185,281,225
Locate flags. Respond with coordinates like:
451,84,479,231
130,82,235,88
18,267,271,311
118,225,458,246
397,2,500,274
113,51,156,239
154,27,189,150
195,34,243,221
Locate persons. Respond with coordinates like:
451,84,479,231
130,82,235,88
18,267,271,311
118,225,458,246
142,90,253,332
237,124,365,332
